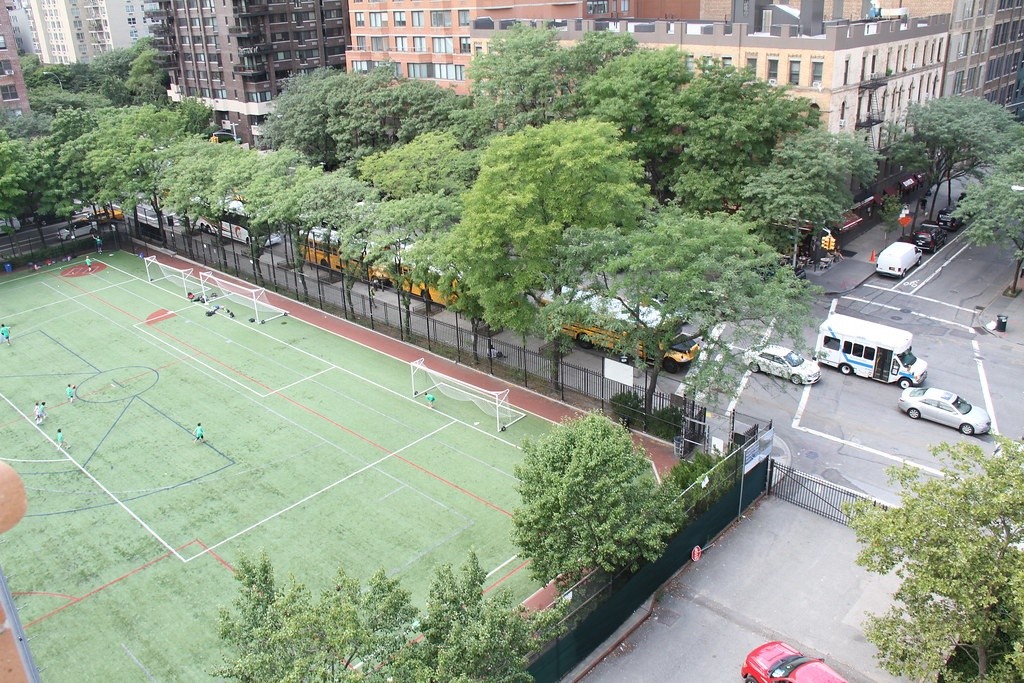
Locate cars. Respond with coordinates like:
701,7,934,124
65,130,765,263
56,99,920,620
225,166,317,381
956,192,981,218
57,219,98,241
898,386,991,436
394,234,416,250
0,215,21,234
36,210,76,226
80,208,124,224
744,344,822,384
741,640,848,683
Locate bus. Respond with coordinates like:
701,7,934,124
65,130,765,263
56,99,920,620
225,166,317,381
539,284,700,373
814,312,928,389
297,225,394,288
188,193,281,247
396,255,466,309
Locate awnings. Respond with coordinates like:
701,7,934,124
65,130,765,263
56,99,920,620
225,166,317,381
874,171,926,206
827,210,864,234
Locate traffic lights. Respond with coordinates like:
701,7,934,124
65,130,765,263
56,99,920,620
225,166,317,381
820,235,837,251
210,137,218,143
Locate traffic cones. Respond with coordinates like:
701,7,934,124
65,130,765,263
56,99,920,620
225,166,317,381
869,248,876,262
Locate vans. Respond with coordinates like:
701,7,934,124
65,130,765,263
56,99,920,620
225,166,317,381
876,241,922,278
758,262,807,290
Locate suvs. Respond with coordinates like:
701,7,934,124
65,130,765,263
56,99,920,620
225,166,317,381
935,206,964,231
912,223,948,253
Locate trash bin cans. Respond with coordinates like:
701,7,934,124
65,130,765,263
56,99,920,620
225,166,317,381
996,314,1008,333
167,215,174,227
3,262,13,272
674,435,684,457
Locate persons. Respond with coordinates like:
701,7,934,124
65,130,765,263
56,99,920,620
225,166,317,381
368,287,377,308
0,324,10,345
85,256,92,271
92,234,102,254
425,392,435,407
403,297,411,310
193,423,204,443
34,402,48,425
487,345,507,359
56,429,64,449
65,384,76,403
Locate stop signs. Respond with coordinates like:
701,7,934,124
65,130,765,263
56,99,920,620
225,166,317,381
691,544,701,562
